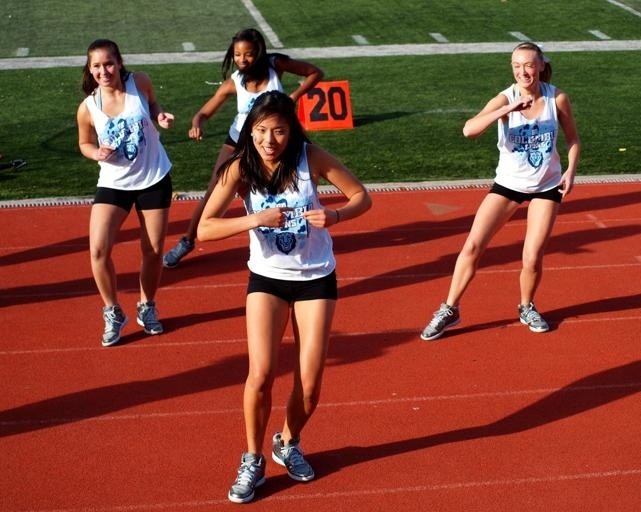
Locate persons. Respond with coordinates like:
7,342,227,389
159,27,328,271
73,37,182,347
194,90,374,504
417,41,583,340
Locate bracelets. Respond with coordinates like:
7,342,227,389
335,209,340,223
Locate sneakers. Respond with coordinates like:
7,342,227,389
102,304,129,347
518,301,550,333
163,237,195,269
137,300,164,335
228,452,268,502
272,432,316,481
420,304,461,340
